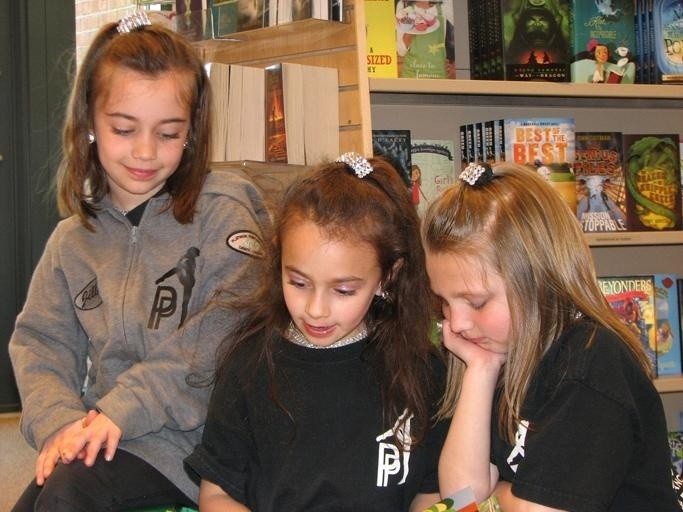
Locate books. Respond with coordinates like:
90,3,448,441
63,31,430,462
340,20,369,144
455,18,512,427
411,140,455,219
371,130,411,189
622,133,682,231
363,0,454,79
633,1,683,85
139,0,342,42
198,63,339,166
595,273,657,377
654,275,681,377
572,1,636,84
459,118,575,218
574,132,626,230
466,2,570,81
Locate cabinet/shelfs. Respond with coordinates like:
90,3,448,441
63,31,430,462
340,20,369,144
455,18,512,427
163,13,375,228
362,13,683,396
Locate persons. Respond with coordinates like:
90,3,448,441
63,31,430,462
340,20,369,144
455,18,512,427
424,162,674,512
6,16,276,512
200,153,457,512
395,0,447,79
